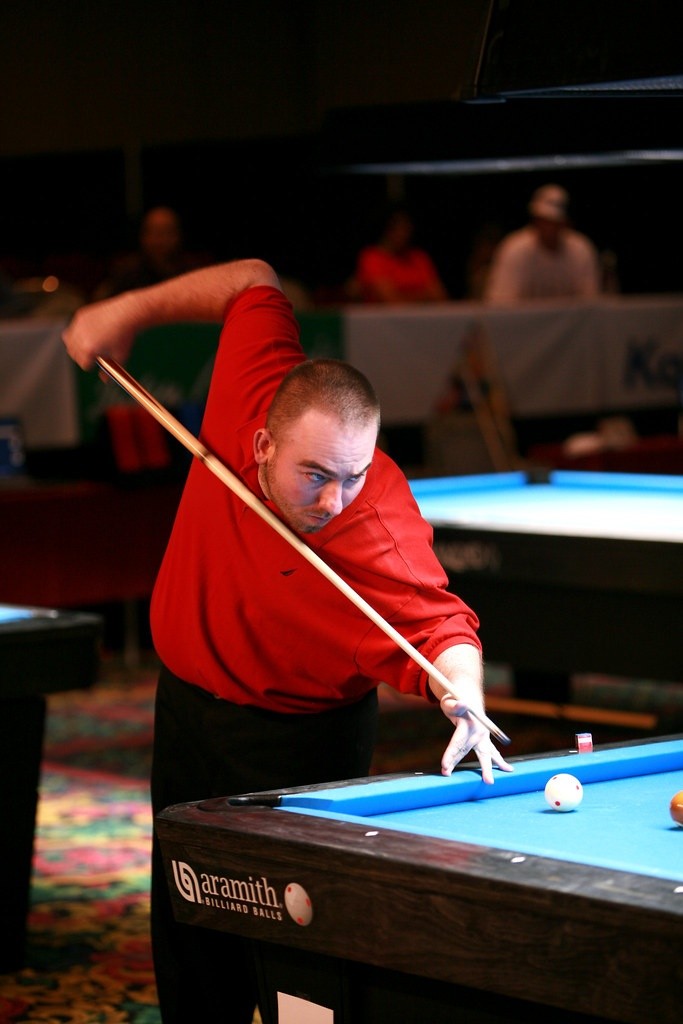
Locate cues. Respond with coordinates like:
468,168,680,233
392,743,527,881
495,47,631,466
96,353,511,747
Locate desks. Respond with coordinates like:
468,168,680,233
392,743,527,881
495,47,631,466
0,603,103,974
153,734,683,1024
0,299,683,455
406,471,683,719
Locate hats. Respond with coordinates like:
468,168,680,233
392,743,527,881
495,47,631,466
529,184,566,222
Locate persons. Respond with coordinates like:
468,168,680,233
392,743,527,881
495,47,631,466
60,207,513,1024
484,183,601,301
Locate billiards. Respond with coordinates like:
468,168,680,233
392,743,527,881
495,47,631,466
285,882,313,926
544,774,584,812
669,790,683,827
